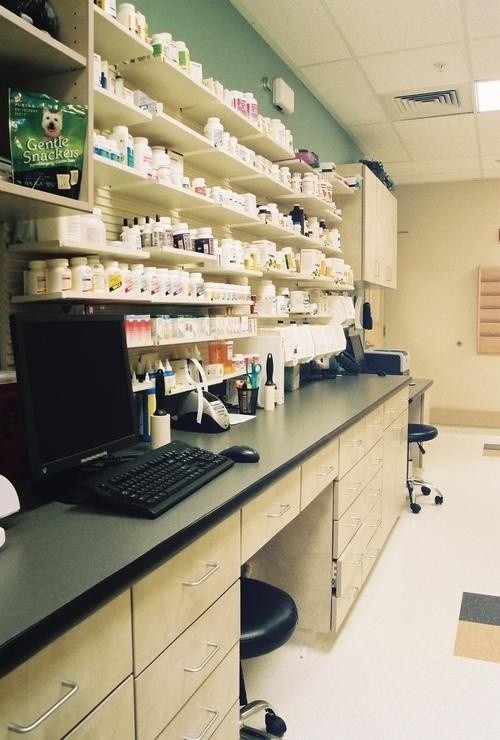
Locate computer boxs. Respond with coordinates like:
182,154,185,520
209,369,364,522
364,349,410,376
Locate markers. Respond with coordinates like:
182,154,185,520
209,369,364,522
236,380,252,414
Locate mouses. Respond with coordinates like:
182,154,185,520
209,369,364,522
219,446,260,463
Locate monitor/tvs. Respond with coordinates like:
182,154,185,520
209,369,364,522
347,334,365,362
9,312,141,505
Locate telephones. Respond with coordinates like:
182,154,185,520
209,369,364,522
335,351,361,374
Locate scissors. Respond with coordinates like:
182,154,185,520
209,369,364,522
245,362,261,389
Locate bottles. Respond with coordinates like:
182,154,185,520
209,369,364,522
208,341,246,374
129,343,203,442
2,1,356,317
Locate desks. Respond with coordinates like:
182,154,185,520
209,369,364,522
408,375,432,469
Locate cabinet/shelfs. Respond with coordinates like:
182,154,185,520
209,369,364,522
364,402,383,581
251,414,364,635
477,266,500,356
243,437,338,563
131,504,242,740
0,580,139,740
0,2,397,403
384,375,409,545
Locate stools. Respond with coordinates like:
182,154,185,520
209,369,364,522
241,575,301,738
406,422,444,513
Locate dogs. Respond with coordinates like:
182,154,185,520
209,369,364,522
40,106,64,142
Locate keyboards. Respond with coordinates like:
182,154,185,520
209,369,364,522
79,437,235,520
